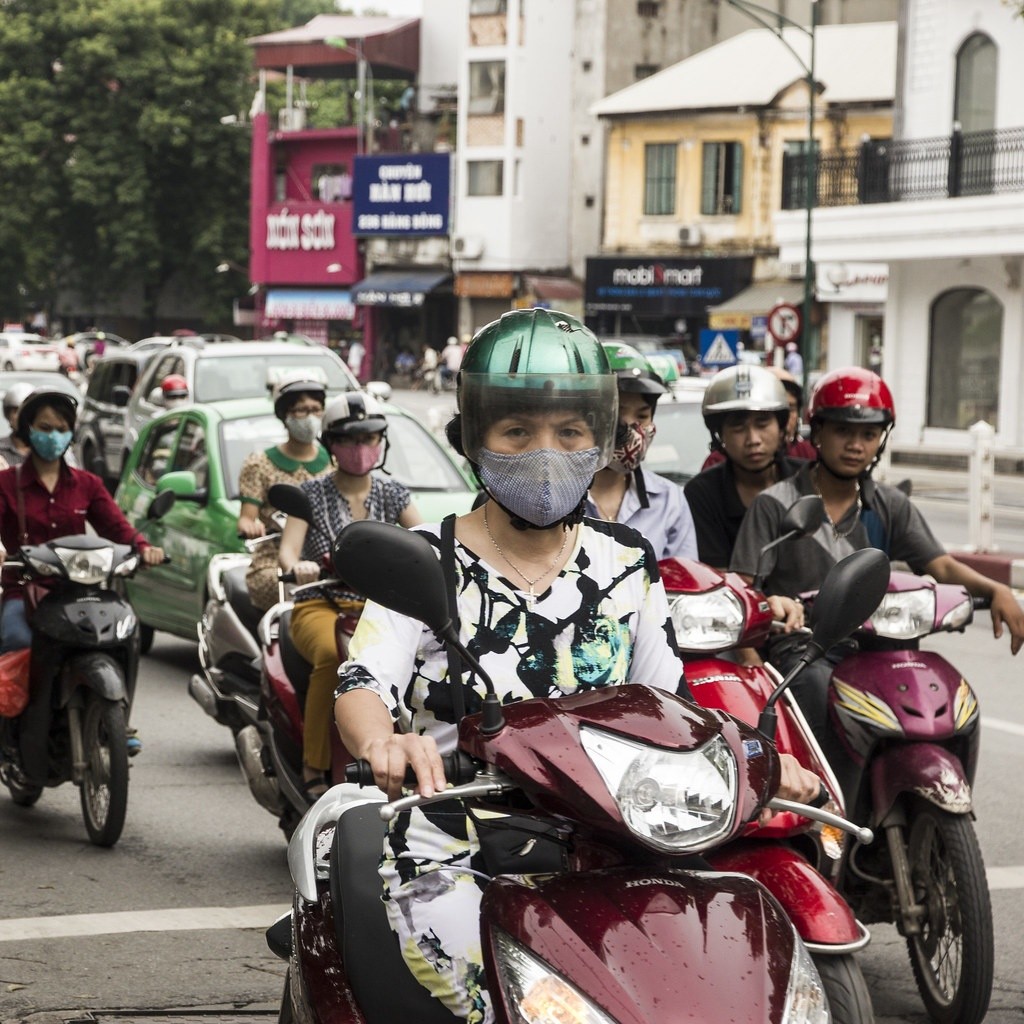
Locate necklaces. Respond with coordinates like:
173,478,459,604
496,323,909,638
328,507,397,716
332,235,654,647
811,469,863,538
484,504,568,594
596,499,614,521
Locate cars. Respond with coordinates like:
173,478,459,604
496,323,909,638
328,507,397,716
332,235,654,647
101,390,485,657
0,323,392,501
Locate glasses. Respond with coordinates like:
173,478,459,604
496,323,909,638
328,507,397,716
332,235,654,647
330,435,381,448
286,406,324,417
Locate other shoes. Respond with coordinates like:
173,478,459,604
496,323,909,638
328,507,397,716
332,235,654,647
298,772,329,805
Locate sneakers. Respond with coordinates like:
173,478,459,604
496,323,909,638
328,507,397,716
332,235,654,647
98,723,142,757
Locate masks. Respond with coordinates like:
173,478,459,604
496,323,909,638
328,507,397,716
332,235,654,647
607,423,656,474
472,446,600,526
330,444,380,476
28,422,73,462
284,416,322,444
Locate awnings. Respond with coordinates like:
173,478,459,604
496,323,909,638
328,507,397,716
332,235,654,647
707,280,806,331
349,268,452,307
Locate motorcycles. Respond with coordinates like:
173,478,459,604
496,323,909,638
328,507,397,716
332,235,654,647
0,525,174,850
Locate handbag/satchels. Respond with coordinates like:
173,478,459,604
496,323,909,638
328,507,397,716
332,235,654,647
18,578,52,618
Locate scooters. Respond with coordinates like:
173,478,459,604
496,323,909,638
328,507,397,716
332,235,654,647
186,481,1001,1024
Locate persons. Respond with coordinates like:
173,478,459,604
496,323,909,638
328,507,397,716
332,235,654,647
395,347,416,374
583,342,804,635
684,362,1024,737
1,382,164,756
419,334,472,394
333,306,821,1024
238,367,339,613
59,339,79,374
90,331,106,356
278,390,425,801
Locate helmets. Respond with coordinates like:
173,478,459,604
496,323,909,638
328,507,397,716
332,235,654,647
702,364,790,430
272,368,328,403
763,366,803,408
599,342,668,393
17,386,78,435
321,391,388,435
803,366,895,430
457,307,612,413
3,382,38,418
